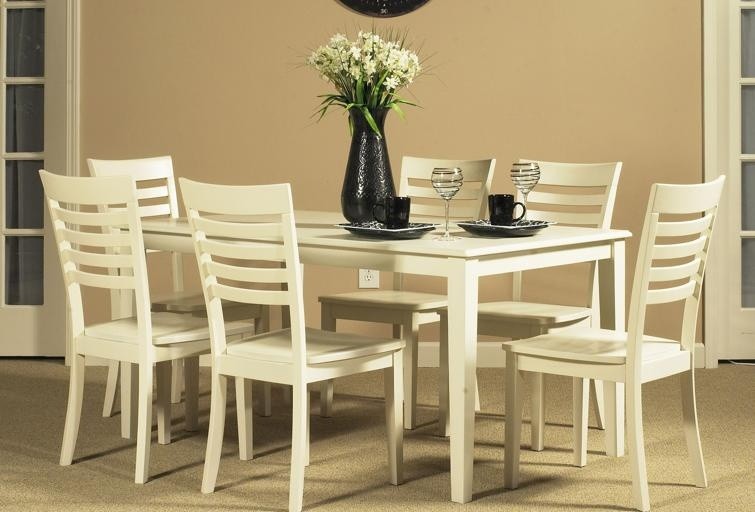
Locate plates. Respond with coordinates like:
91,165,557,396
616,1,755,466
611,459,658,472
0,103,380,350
335,222,440,238
453,220,558,237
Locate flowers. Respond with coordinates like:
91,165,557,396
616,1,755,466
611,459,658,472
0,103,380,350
292,13,441,137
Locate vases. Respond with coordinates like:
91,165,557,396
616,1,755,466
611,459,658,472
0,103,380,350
339,104,400,226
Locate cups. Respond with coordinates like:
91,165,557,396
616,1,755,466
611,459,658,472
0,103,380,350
488,194,525,225
372,196,412,228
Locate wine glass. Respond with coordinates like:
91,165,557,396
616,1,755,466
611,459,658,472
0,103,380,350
510,161,542,219
431,166,463,241
